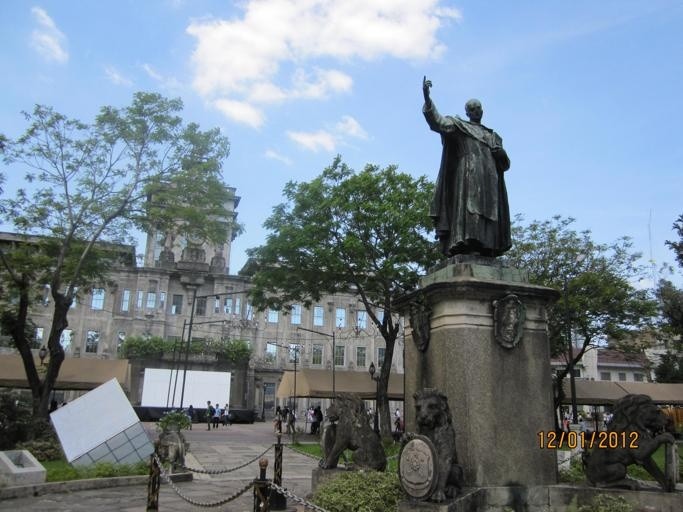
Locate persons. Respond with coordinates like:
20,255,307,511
184,401,233,431
422,75,512,257
367,408,401,434
272,406,323,436
562,409,614,434
